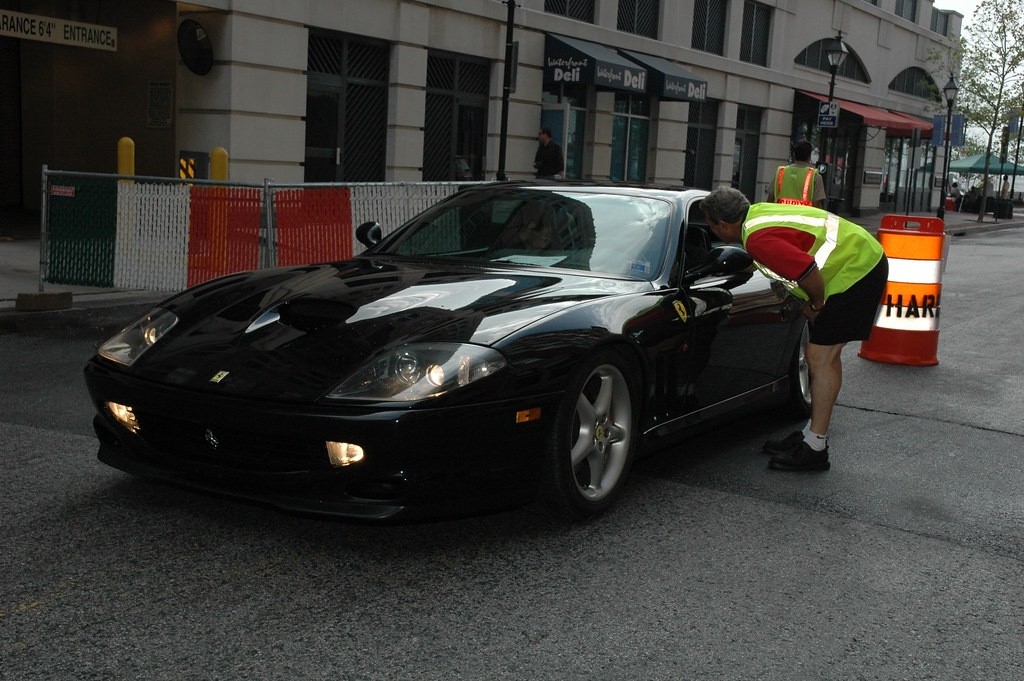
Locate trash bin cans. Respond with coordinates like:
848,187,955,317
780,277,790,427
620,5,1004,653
829,196,845,217
995,199,1013,218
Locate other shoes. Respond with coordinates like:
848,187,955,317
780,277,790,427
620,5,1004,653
770,441,830,473
764,431,806,455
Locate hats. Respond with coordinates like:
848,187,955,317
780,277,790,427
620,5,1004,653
952,183,958,187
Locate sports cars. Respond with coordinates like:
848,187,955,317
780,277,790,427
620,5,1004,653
82,180,818,523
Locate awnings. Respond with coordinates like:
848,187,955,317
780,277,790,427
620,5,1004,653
543,33,709,107
799,90,933,130
886,110,951,140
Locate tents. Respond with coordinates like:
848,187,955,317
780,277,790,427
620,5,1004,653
949,153,1024,175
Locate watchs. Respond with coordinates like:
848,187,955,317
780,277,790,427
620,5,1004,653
808,303,818,312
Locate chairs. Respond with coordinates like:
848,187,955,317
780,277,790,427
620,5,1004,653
678,226,711,263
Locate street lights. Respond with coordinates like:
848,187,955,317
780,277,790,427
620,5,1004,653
1009,80,1024,201
936,69,960,219
813,28,850,184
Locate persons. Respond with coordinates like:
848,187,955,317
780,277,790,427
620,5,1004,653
967,186,978,200
534,127,565,180
767,140,826,208
1001,176,1009,198
434,154,471,181
951,183,962,199
698,184,888,473
